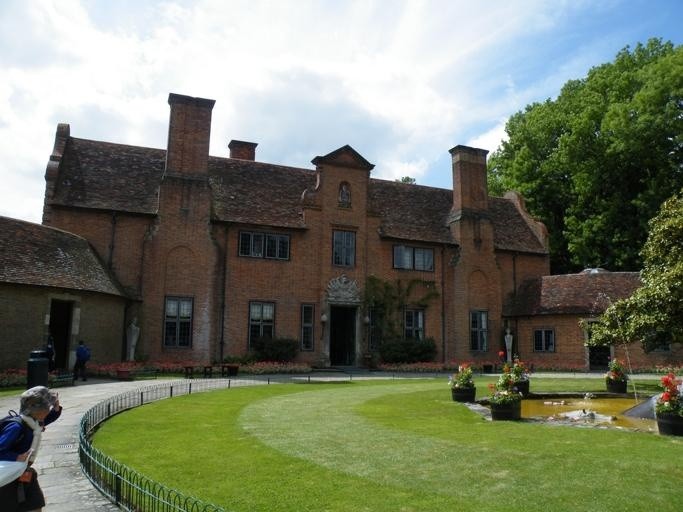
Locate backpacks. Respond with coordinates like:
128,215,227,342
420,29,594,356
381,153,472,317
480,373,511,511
0,410,29,457
80,347,89,360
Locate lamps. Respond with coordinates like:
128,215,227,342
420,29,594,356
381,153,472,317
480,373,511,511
320,314,328,340
363,316,370,341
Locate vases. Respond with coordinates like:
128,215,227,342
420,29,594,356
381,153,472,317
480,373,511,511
452,387,476,403
506,376,529,395
656,413,683,437
490,399,521,420
606,376,627,393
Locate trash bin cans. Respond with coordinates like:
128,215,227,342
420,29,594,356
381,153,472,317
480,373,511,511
27,351,49,390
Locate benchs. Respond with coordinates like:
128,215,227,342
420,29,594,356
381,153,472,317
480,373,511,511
128,369,159,379
48,368,75,387
221,364,239,377
185,365,213,378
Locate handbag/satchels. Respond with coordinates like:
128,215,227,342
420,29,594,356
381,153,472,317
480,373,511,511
0,466,45,511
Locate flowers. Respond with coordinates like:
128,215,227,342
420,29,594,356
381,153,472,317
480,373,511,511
498,351,532,383
607,356,628,382
483,381,523,404
448,363,474,391
654,373,683,416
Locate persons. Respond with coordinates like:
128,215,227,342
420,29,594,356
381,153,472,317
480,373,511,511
73,340,90,381
0,385,64,511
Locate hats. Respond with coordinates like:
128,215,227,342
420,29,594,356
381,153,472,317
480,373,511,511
19,386,57,416
